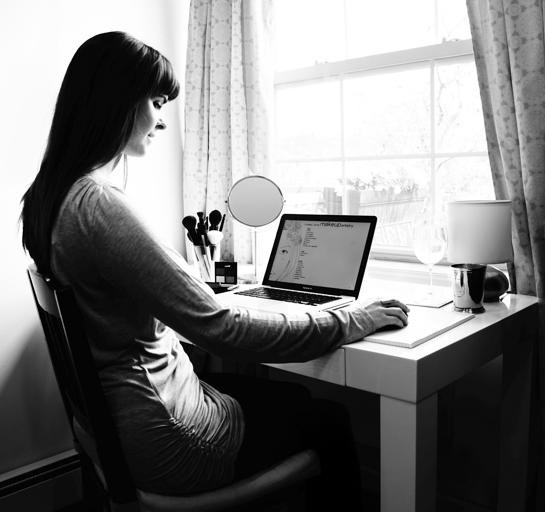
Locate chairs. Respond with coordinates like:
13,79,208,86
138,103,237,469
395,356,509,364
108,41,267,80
26,263,320,510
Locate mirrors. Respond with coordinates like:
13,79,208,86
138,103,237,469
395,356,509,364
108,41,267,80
225,175,286,278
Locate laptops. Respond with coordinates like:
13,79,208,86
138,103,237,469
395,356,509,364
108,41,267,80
215,214,376,316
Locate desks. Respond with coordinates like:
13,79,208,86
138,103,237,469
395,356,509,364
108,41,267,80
174,278,541,512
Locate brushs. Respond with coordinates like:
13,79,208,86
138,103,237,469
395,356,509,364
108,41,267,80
180,209,227,280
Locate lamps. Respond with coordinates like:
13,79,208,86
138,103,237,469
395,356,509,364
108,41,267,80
446,198,517,317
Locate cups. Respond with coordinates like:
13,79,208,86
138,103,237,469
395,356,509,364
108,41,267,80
451,262,487,315
194,245,221,282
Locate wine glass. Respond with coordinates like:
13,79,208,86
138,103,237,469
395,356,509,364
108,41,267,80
413,215,448,301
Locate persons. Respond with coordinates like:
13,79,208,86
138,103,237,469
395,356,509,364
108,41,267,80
19,31,409,510
